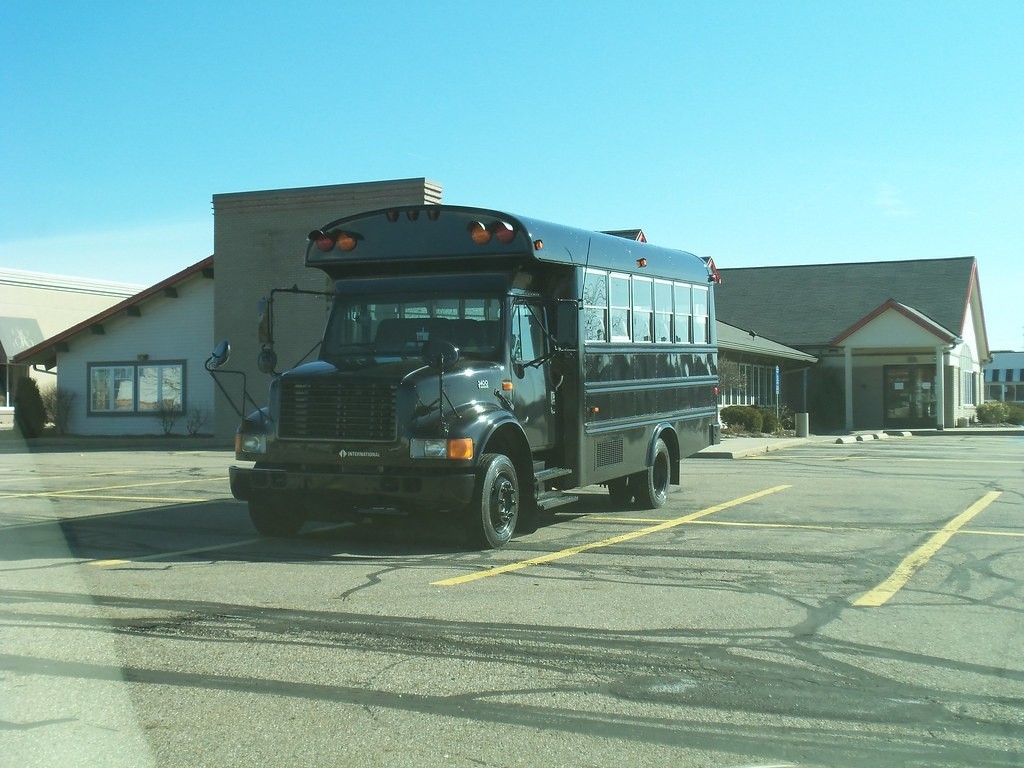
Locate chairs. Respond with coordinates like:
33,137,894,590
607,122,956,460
376,318,502,351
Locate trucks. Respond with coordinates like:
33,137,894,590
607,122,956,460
203,203,722,551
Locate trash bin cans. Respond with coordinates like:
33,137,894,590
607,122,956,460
794,412,809,437
